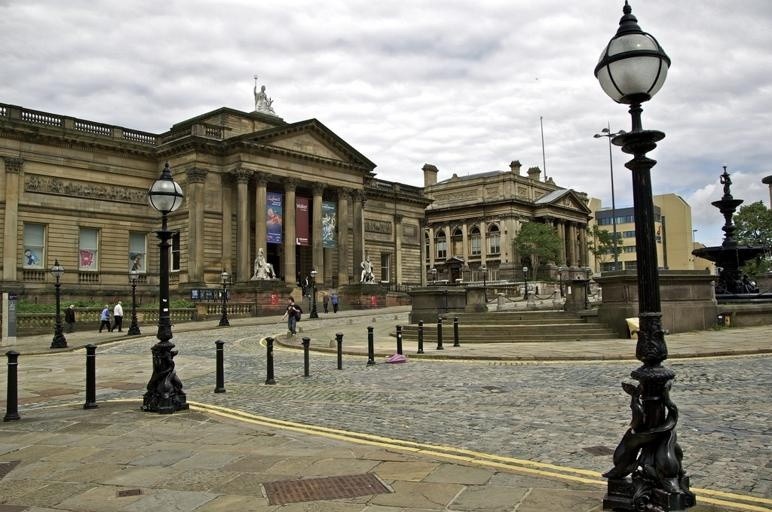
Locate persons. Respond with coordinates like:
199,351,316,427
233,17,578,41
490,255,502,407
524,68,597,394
254,84,275,116
65,304,76,333
281,296,302,336
252,248,276,278
322,292,338,314
359,255,376,283
296,268,318,298
98,300,124,333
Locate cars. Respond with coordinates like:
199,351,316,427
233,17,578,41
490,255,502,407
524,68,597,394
520,283,542,295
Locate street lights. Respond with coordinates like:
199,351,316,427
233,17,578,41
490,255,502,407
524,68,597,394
587,0,702,512
480,263,490,303
47,257,70,350
125,264,143,336
656,224,669,269
558,266,564,296
215,270,231,327
522,266,529,300
692,229,698,249
592,121,629,270
432,268,437,285
141,158,192,416
307,269,318,319
